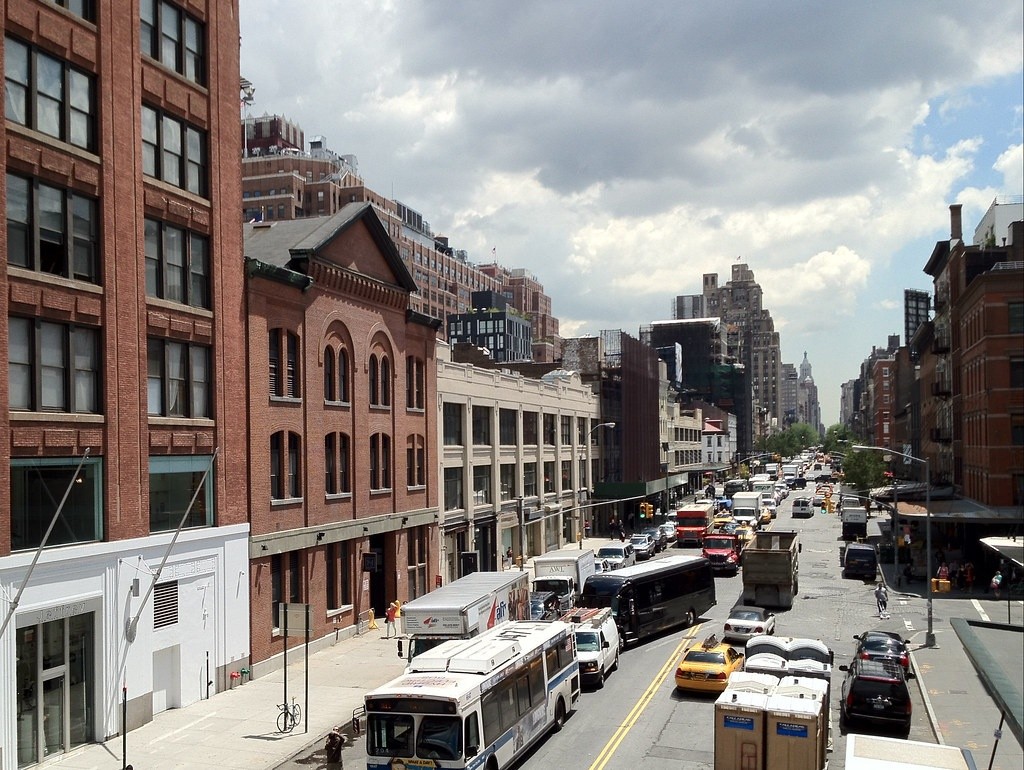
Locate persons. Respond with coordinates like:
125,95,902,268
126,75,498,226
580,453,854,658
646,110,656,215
385,601,408,639
936,562,1018,598
507,547,513,569
585,520,591,537
903,562,911,584
325,726,345,770
875,583,888,612
609,519,626,542
836,502,841,516
865,499,882,516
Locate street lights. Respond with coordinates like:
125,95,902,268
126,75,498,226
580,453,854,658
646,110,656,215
852,444,937,647
579,422,616,549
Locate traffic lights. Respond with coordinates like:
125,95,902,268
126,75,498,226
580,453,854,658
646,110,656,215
640,504,646,519
828,501,836,513
646,504,654,519
820,501,828,514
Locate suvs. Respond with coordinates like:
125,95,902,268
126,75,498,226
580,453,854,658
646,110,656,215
530,591,562,622
596,542,636,571
837,656,912,739
628,534,656,559
594,558,612,574
792,496,815,517
844,545,878,581
641,527,669,553
559,607,621,688
667,512,679,529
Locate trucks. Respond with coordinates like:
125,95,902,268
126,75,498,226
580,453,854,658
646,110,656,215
712,635,833,769
398,570,530,670
846,734,968,770
841,498,870,535
699,533,749,576
730,492,763,531
533,549,595,616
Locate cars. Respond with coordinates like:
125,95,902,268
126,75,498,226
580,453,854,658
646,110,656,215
675,637,747,697
852,630,911,676
693,448,840,531
722,606,776,641
660,523,678,543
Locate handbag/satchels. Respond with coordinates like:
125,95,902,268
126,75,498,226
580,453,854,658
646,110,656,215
385,616,388,623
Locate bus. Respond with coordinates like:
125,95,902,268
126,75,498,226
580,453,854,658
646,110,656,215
579,555,718,646
352,620,579,769
676,504,715,545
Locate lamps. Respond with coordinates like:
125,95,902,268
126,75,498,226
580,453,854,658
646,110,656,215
130,579,139,597
